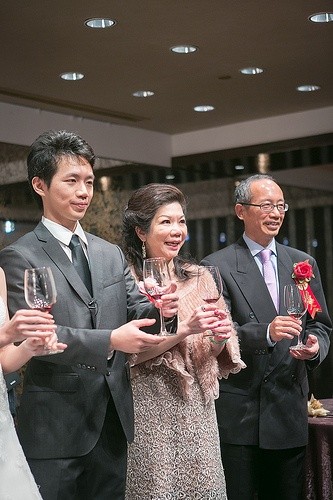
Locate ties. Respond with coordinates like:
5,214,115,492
259,249,279,315
68,235,93,298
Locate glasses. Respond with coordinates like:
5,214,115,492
240,202,288,212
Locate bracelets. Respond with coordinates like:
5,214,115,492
209,336,228,344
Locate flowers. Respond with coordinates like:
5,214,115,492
307,393,331,419
290,258,316,283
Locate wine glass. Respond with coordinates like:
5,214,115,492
197,265,223,336
142,257,178,337
24,266,65,356
283,283,313,350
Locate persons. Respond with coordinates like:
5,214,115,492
198,175,333,500
0,130,181,500
121,184,248,500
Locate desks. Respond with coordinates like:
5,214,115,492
305,399,333,500
